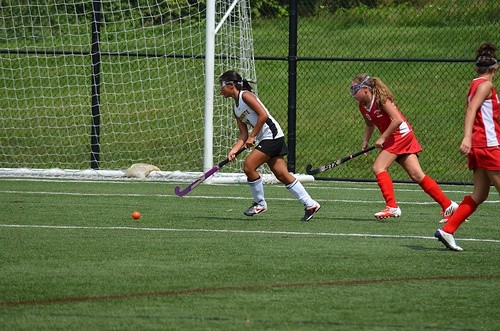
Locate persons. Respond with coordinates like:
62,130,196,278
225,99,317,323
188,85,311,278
434,43,500,251
219,70,322,222
350,73,459,223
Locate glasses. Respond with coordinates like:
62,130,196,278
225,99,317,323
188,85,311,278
350,83,372,95
221,81,234,87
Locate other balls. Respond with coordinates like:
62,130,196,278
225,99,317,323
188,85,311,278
132,212,141,220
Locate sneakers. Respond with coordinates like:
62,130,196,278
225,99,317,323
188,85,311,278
434,229,463,251
374,205,402,219
244,203,267,216
439,200,461,223
300,201,321,221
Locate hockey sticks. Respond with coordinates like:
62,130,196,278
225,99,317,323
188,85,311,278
174,140,256,196
306,144,386,176
